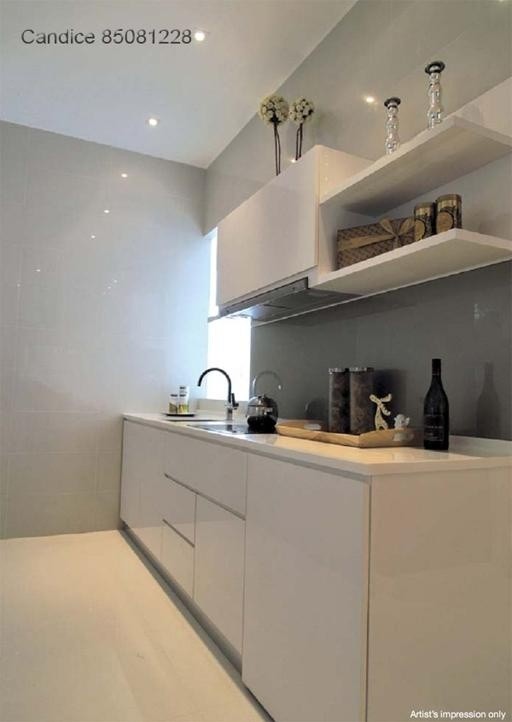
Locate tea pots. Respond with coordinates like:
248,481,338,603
244,394,279,430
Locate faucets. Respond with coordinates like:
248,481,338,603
197,367,239,420
251,369,284,397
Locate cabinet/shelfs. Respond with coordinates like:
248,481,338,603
307,116,512,297
205,144,362,327
120,413,512,721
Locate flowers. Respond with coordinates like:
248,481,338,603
259,94,315,177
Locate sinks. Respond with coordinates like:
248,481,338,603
164,418,224,423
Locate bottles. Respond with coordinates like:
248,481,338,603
327,365,374,436
413,192,463,241
421,356,451,451
168,385,189,415
